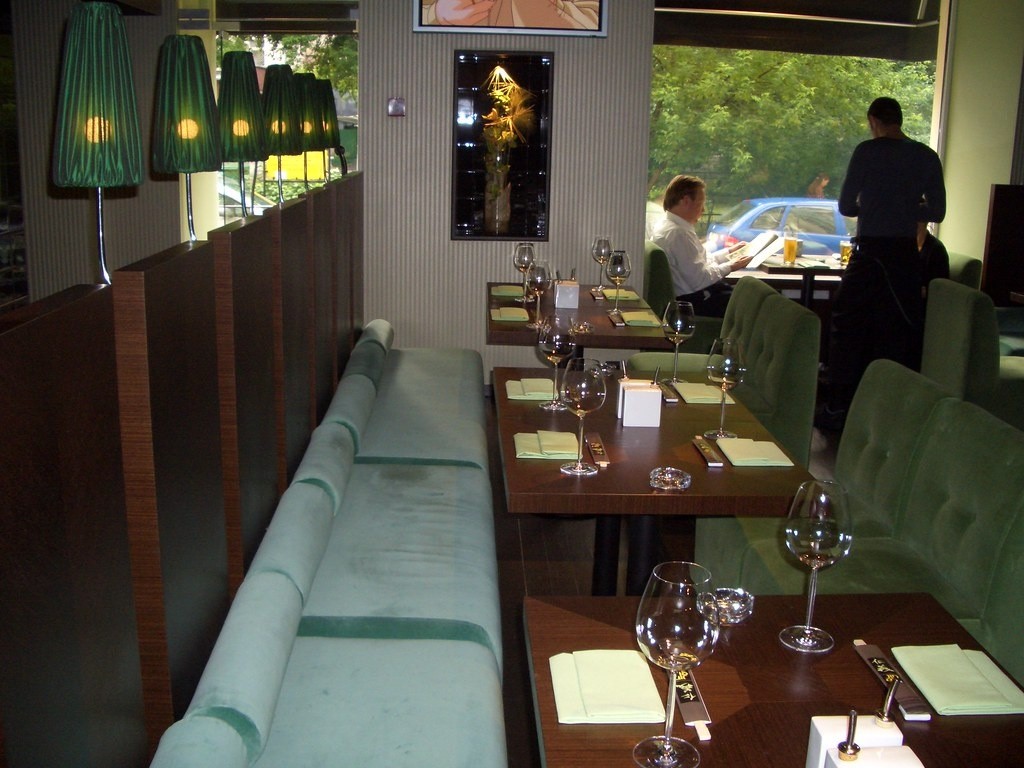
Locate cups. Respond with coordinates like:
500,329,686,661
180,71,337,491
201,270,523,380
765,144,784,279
796,239,803,257
840,240,854,264
784,231,798,264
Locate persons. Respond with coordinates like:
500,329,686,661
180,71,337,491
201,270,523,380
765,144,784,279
650,173,754,318
818,97,946,432
807,173,829,198
911,196,951,336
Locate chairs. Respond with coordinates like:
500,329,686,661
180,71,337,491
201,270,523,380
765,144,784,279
926,250,1023,426
641,240,723,355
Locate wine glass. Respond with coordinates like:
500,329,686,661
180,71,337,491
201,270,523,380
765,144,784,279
633,562,720,768
538,314,576,411
606,252,631,314
704,338,746,441
560,358,606,476
513,243,535,301
780,481,854,652
591,237,612,291
661,301,697,386
526,260,551,329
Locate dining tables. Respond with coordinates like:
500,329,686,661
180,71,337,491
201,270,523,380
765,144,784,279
524,572,1024,768
761,253,849,310
486,281,673,365
488,364,827,590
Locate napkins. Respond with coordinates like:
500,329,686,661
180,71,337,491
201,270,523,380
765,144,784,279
795,258,829,270
489,307,530,320
890,644,1024,714
603,287,641,300
513,430,585,460
490,284,524,296
716,438,798,467
504,378,559,399
548,650,666,723
672,382,736,404
620,310,661,327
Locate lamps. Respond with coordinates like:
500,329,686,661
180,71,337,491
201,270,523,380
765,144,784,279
291,73,326,193
152,35,222,240
316,77,342,181
260,63,302,204
219,50,268,215
52,2,143,282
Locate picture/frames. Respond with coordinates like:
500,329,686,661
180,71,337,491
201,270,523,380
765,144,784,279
413,0,609,37
452,46,555,241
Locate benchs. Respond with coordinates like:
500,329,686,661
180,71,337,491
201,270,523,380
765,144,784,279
633,278,822,591
685,358,1022,681
151,318,509,768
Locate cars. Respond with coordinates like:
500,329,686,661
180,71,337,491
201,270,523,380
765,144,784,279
705,196,859,255
218,172,276,227
